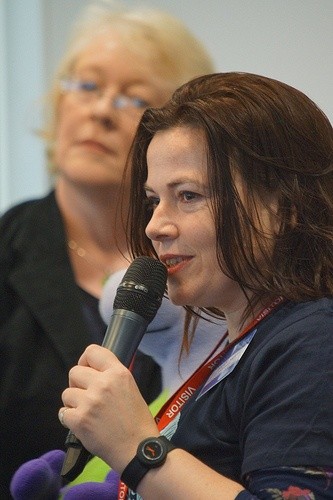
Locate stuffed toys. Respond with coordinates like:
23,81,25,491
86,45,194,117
9,268,229,500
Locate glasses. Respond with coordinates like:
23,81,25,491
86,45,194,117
64,71,161,117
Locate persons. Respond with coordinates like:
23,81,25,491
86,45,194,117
58,71,333,500
0,6,215,500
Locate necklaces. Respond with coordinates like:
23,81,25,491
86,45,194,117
117,295,284,500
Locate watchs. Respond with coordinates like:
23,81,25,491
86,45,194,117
120,435,177,492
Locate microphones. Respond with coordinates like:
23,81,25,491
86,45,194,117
58,255,168,487
